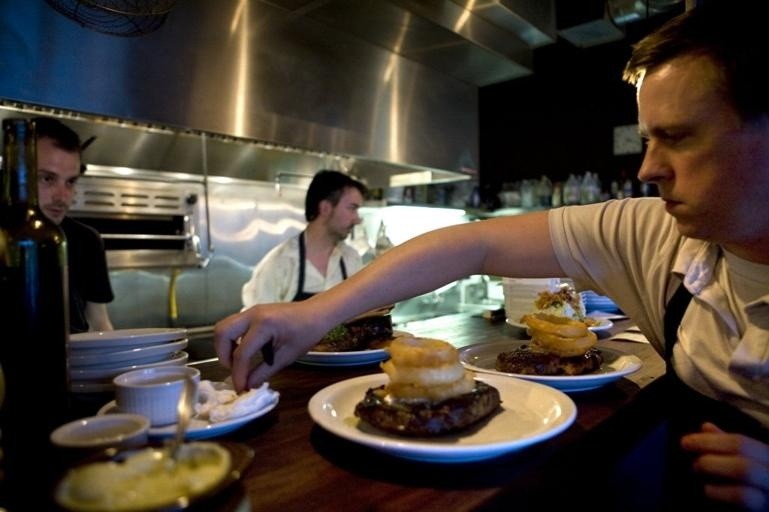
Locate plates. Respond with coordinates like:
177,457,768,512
50,412,150,448
309,373,578,463
97,377,280,441
505,314,614,331
62,326,194,392
53,439,255,512
296,329,413,366
456,340,644,391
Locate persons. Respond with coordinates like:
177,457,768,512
34,113,114,333
239,169,365,316
212,0,769,512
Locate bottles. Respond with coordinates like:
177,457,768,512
520,172,633,207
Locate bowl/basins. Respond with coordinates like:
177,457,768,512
116,360,203,425
